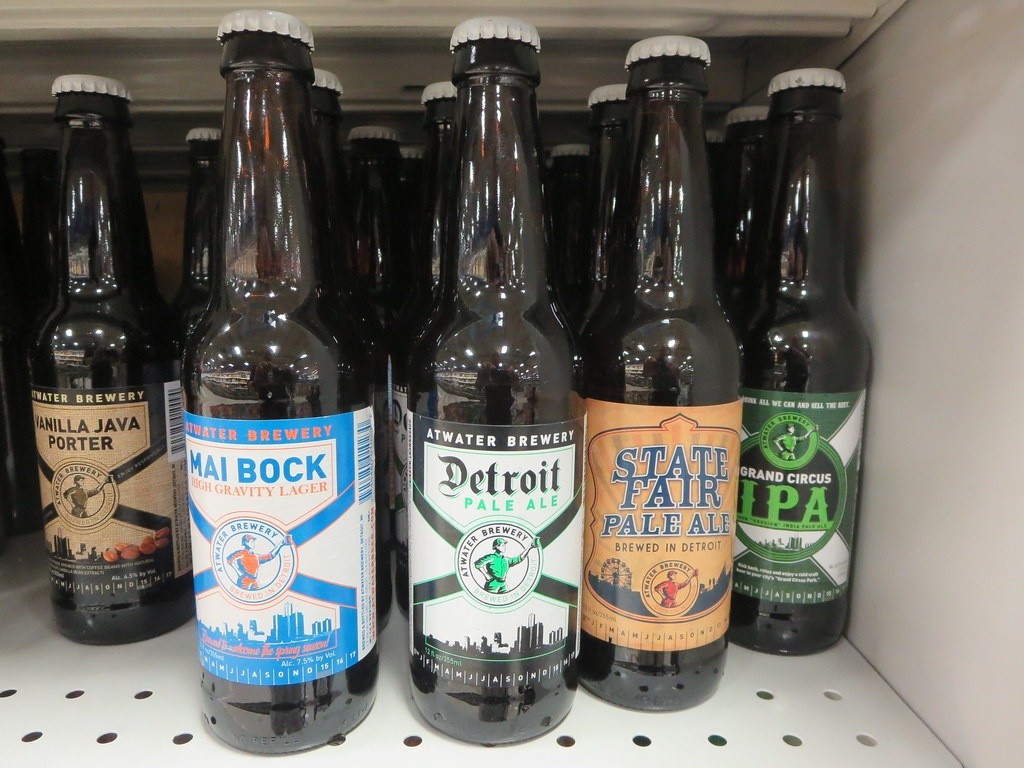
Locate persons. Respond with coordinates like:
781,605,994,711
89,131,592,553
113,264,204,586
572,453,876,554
642,344,681,406
183,361,203,417
91,347,118,388
777,333,811,393
476,351,520,425
252,347,293,420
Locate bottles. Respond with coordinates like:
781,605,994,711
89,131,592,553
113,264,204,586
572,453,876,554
345,127,411,298
310,68,390,639
18,147,67,309
729,68,874,656
402,147,419,194
727,105,768,346
167,129,224,349
581,83,626,328
183,8,390,752
0,138,43,536
705,128,728,213
390,82,458,616
551,143,589,318
29,74,194,646
579,37,742,710
413,15,586,744
